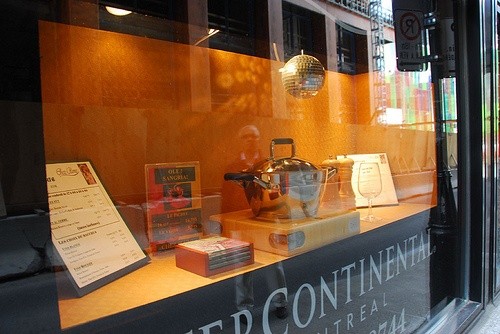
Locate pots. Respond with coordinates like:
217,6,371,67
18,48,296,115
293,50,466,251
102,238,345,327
223,136,338,221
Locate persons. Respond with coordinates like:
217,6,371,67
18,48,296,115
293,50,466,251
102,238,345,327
219,125,289,324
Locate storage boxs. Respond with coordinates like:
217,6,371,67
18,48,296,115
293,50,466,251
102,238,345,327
175,236,254,278
210,205,362,254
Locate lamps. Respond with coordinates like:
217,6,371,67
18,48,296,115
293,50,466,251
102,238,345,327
280,0,325,97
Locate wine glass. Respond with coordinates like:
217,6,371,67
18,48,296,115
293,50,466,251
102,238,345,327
356,165,384,223
